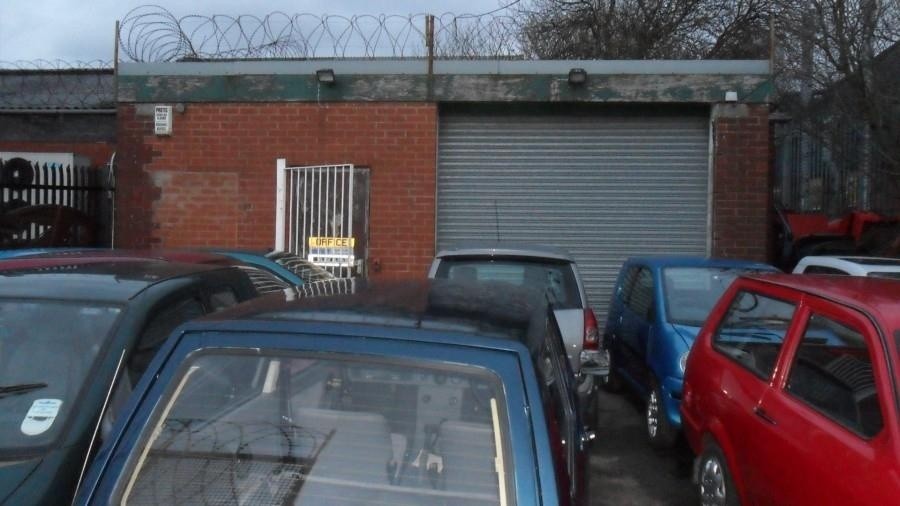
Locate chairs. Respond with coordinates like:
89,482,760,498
522,265,552,290
268,404,501,506
453,265,477,284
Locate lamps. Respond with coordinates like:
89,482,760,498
567,68,587,87
315,68,336,86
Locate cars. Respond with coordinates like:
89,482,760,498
70,272,590,506
683,276,899,505
1,255,267,504
602,256,848,453
791,255,900,345
1,246,334,296
427,245,610,412
